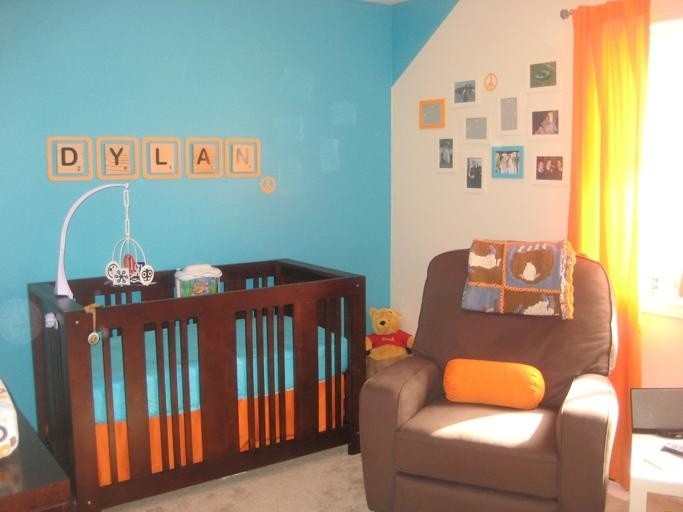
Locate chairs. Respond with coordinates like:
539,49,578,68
357,249,620,510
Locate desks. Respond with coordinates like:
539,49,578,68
0,373,73,511
629,431,683,512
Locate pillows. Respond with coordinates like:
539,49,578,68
443,357,547,410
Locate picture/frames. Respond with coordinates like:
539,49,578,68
491,145,525,179
419,99,446,129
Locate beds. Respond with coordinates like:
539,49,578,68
26,258,366,511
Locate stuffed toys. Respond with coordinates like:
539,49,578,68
365,307,415,360
